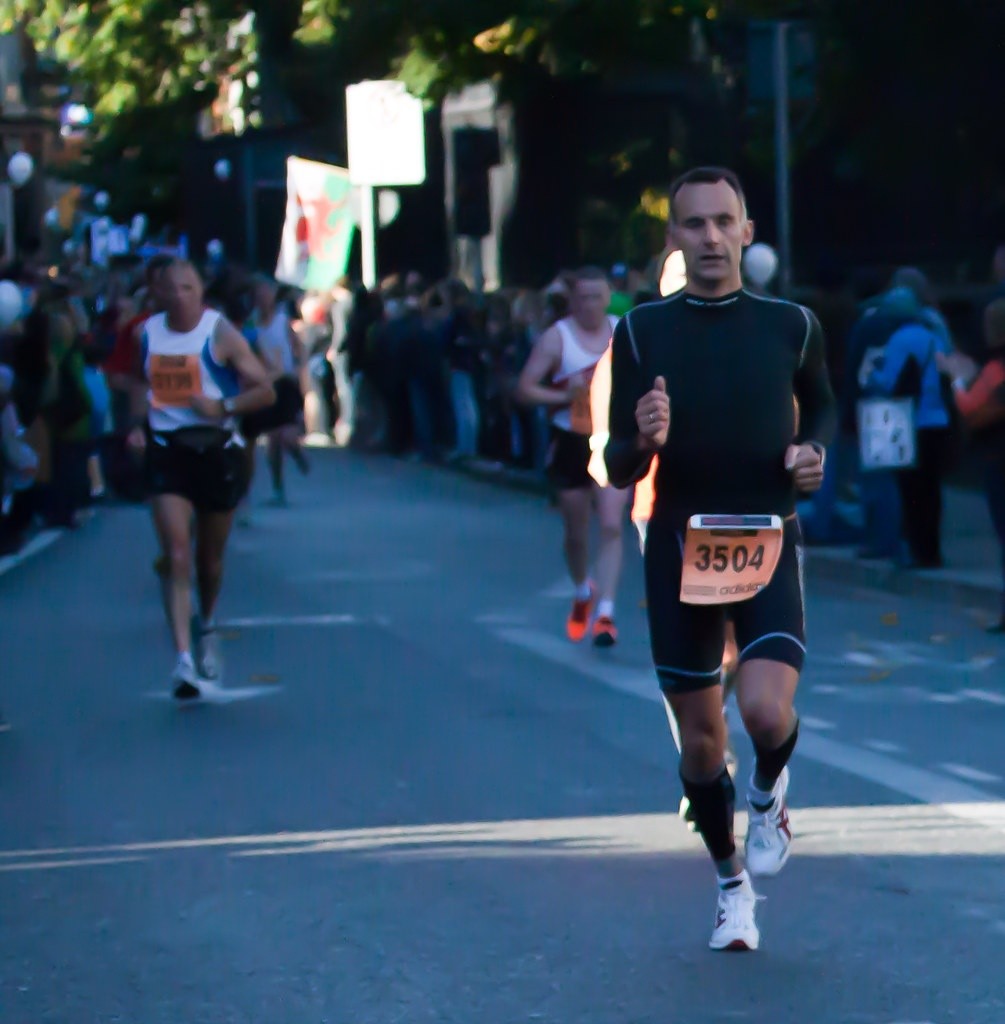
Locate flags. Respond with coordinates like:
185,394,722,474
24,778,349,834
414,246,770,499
274,158,355,291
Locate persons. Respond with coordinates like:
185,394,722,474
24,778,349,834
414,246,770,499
292,264,580,469
0,249,196,574
938,298,1005,632
603,168,838,952
125,258,276,699
252,282,310,508
589,248,800,831
516,266,627,646
799,268,952,568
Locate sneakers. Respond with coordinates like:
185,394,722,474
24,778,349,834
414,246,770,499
709,882,767,951
173,661,201,698
593,617,617,645
191,613,220,678
567,579,596,641
744,758,791,879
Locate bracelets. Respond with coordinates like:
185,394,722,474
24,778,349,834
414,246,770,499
951,379,963,389
589,432,609,451
802,439,825,470
222,397,234,415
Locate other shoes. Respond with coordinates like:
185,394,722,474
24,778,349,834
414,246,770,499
260,494,287,507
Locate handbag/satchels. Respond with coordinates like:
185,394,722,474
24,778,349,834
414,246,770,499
858,393,914,468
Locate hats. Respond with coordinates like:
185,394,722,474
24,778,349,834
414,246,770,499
883,289,916,315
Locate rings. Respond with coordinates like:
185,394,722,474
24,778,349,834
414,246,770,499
650,414,654,422
